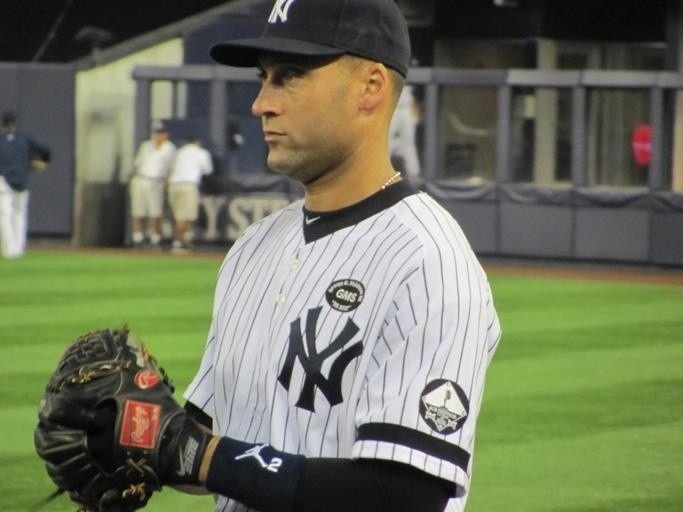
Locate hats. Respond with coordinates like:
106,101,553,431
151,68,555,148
209,0,411,78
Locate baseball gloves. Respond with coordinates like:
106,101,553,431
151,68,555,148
33,326,214,510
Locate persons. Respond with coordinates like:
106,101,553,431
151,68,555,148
127,118,177,247
0,110,50,266
32,0,505,511
168,132,213,255
